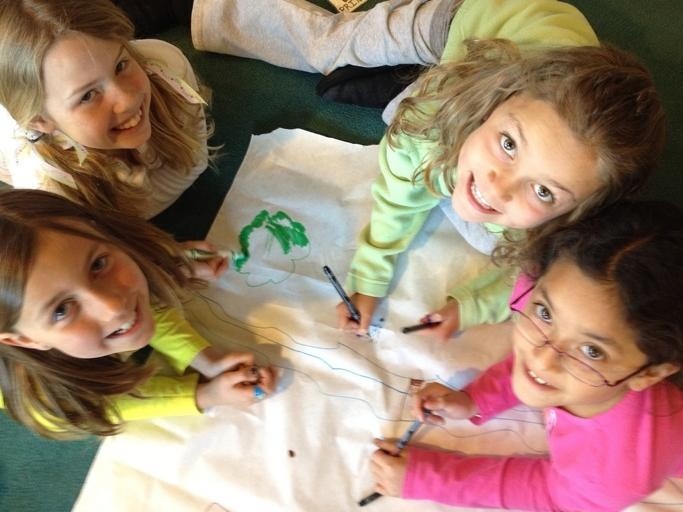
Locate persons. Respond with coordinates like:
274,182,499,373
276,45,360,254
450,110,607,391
0,1,229,282
1,187,278,441
369,178,682,512
190,1,670,345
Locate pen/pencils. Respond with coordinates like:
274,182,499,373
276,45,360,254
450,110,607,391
184,246,246,261
401,321,435,334
322,265,360,324
238,363,274,396
392,410,431,457
359,492,379,507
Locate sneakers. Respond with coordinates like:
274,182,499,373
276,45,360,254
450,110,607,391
316,64,419,109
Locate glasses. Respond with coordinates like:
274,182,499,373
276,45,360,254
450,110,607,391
508,285,658,387
